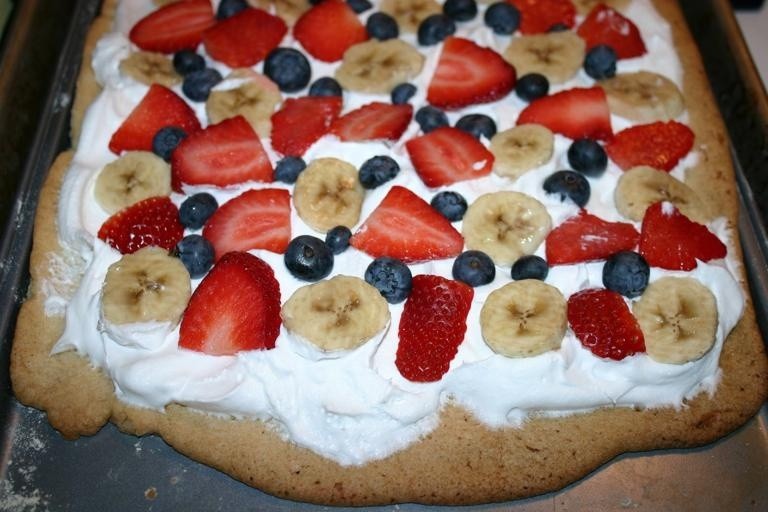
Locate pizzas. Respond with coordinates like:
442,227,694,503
10,0,768,507
